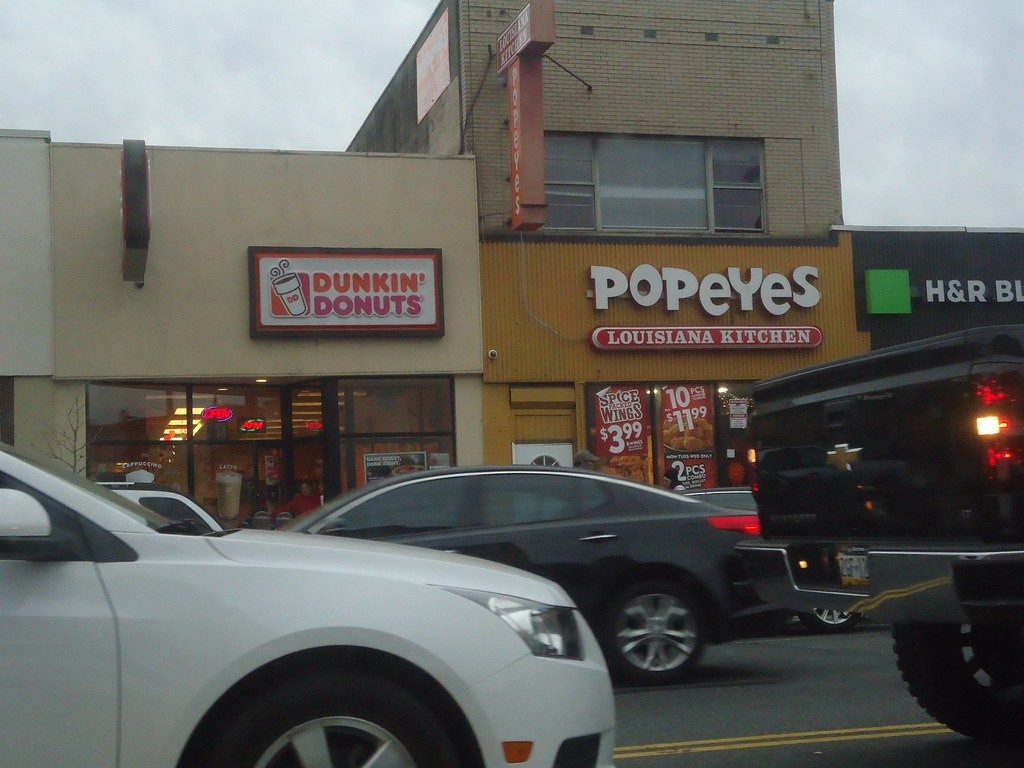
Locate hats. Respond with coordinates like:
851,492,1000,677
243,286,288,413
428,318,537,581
664,469,679,483
573,450,599,463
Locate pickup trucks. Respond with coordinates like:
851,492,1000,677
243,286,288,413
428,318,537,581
731,320,1024,748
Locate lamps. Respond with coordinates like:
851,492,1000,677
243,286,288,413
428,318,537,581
159,407,207,441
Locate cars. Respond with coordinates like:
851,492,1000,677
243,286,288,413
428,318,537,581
674,485,859,633
0,441,617,768
279,462,764,689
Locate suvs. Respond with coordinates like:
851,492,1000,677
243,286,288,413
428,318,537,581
91,481,224,534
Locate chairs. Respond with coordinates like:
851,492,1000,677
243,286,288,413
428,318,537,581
275,512,293,528
252,511,271,530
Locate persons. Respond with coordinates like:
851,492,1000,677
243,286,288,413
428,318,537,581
663,468,686,492
264,491,281,514
574,449,601,471
272,479,322,516
